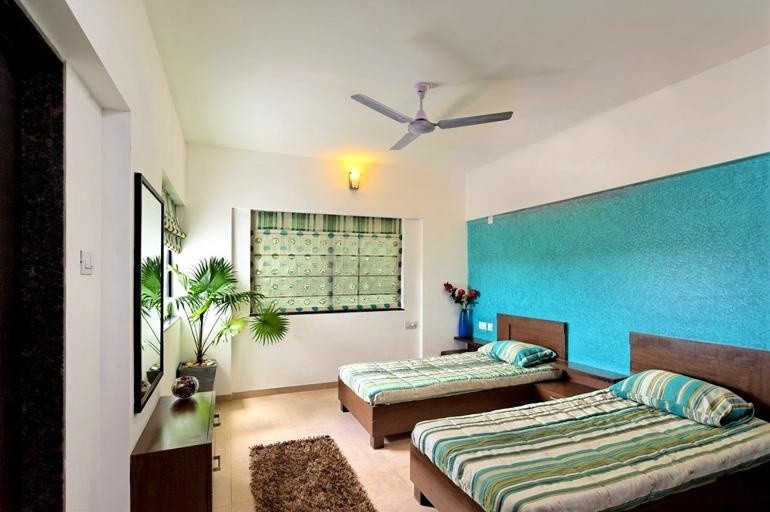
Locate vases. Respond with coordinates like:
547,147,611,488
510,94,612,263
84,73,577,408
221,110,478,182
457,307,470,338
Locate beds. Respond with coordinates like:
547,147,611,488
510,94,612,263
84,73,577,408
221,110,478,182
409,332,770,511
337,313,569,448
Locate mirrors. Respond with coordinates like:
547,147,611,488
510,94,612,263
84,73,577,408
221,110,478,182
132,171,165,415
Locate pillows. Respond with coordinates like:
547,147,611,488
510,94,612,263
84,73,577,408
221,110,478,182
610,367,755,428
476,340,558,369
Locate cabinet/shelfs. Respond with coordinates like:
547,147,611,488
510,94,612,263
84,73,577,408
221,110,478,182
132,390,220,511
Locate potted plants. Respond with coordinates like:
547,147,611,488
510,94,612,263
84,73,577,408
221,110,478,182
166,255,290,392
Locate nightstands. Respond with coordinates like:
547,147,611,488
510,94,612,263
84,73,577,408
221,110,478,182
530,361,625,403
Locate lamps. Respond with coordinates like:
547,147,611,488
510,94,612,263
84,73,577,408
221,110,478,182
340,152,366,191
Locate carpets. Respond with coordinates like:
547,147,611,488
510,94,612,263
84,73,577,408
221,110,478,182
250,434,377,511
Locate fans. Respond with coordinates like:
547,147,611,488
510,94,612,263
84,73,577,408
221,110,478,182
348,81,513,152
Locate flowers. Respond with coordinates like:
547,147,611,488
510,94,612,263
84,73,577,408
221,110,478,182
443,281,480,309
